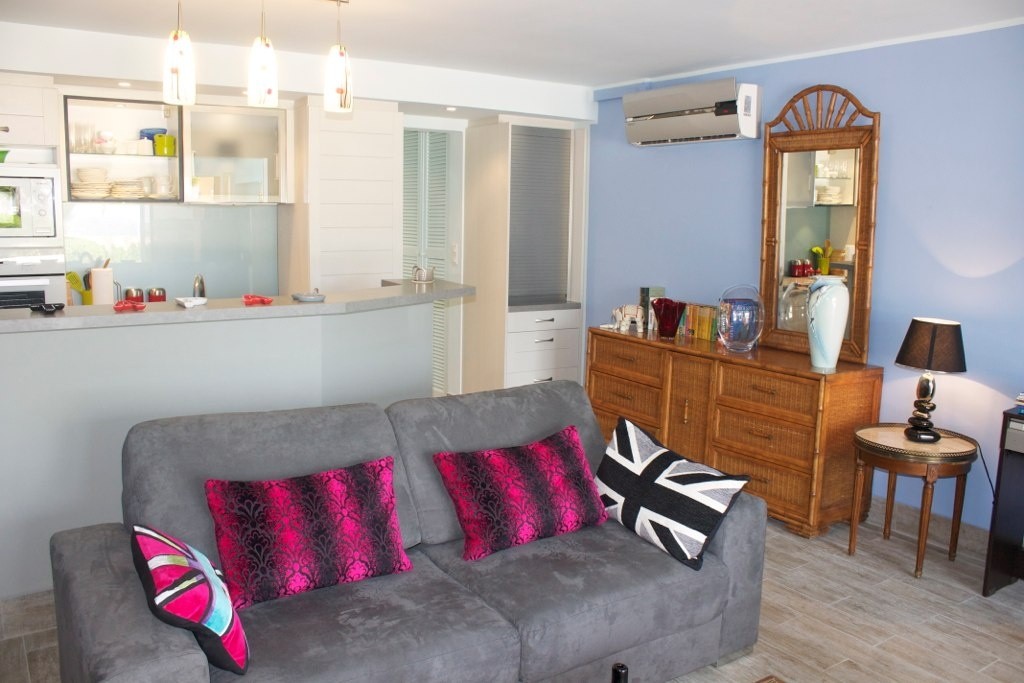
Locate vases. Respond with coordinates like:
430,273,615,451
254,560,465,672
806,275,850,368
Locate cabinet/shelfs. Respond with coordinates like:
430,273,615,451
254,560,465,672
786,148,859,208
463,114,885,541
0,70,403,298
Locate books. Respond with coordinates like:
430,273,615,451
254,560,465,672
640,287,720,342
1015,392,1024,413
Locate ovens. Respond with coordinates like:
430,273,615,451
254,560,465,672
0,248,65,310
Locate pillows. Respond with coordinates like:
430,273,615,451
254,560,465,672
130,524,250,676
433,426,609,562
594,416,752,571
203,455,415,613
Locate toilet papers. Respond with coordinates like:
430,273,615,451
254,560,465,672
844,244,856,278
89,266,113,305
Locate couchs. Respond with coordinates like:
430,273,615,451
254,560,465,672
50,375,767,683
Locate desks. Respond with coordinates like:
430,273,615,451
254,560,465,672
849,423,978,578
983,407,1024,598
783,269,848,288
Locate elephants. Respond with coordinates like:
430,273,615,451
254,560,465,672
611,304,644,332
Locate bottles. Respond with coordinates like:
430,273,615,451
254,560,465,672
716,285,765,353
805,275,850,369
611,663,628,683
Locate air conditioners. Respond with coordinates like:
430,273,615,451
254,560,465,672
622,76,762,147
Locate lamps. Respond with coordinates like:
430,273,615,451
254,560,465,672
894,318,967,443
163,0,353,112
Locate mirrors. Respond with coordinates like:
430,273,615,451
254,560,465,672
759,84,881,367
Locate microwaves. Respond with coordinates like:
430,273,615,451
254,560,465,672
0,177,55,238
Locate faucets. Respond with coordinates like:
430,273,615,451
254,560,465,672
193,275,206,299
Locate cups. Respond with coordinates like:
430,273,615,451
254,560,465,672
650,297,688,341
83,291,92,305
140,175,175,194
95,128,174,156
149,288,166,302
214,175,230,202
126,289,143,302
68,123,95,154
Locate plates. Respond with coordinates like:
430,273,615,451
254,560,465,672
148,194,177,200
70,166,146,199
293,293,326,301
192,175,214,201
